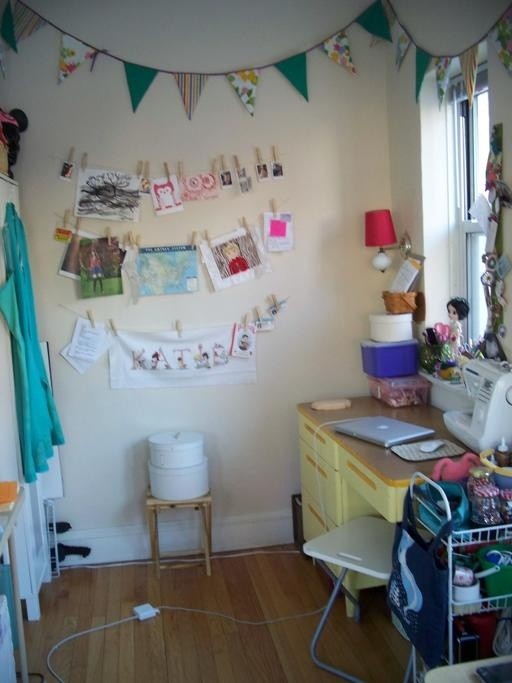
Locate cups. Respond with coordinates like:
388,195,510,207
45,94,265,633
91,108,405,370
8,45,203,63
453,575,481,601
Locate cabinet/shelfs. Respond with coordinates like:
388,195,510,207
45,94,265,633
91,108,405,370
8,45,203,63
0,173,54,623
407,470,511,683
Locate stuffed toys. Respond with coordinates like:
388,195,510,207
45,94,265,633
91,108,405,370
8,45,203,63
431,452,484,482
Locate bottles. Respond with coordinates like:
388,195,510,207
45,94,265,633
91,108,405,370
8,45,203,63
490,455,498,466
495,436,510,467
433,357,442,377
468,489,512,525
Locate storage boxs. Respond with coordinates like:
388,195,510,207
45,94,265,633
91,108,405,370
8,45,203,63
358,338,422,375
368,314,413,342
367,374,430,409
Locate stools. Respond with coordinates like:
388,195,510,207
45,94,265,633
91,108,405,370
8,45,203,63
145,484,213,577
302,514,410,683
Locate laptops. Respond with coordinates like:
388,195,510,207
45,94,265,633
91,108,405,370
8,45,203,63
334,415,435,448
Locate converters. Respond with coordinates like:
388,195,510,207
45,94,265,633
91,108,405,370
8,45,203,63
132,602,156,621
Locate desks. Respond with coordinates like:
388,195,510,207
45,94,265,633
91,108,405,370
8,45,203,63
0,487,30,682
426,654,512,682
297,387,512,620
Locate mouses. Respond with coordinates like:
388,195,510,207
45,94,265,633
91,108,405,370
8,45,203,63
419,440,445,452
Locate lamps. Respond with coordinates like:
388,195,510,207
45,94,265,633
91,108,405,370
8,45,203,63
365,209,415,272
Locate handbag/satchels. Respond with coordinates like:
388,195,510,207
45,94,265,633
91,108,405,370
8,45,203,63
389,522,447,668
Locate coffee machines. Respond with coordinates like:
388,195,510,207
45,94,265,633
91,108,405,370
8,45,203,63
442,357,511,455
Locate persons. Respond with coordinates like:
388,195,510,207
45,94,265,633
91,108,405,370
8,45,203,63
430,295,470,364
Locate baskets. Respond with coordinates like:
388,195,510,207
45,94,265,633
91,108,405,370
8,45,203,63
383,290,418,314
